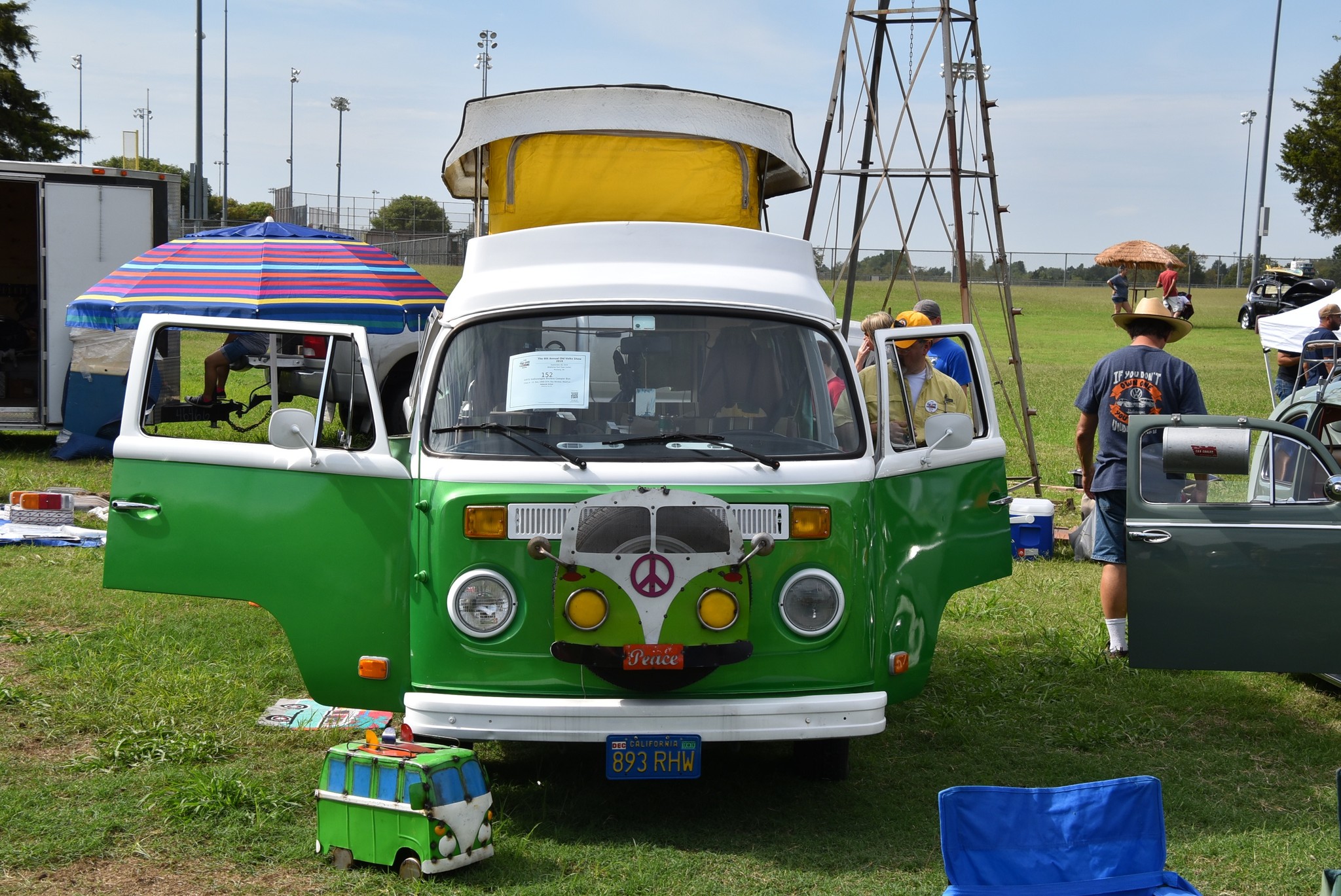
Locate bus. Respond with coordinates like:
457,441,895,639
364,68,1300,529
315,738,496,882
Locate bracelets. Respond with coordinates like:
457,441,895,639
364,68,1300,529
221,343,224,347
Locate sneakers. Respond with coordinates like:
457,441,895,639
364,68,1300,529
184,393,213,408
216,392,227,398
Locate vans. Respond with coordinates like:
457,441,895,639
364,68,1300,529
101,220,1012,784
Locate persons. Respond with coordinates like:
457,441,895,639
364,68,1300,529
1170,288,1191,318
1156,261,1183,318
1272,349,1306,402
814,300,975,453
1073,297,1211,661
1107,265,1133,328
1302,303,1341,389
185,331,270,408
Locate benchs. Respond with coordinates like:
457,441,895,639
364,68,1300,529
1128,287,1155,302
489,397,699,425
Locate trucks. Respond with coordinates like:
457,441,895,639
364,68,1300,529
0,161,426,440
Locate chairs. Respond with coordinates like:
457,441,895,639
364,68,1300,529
930,769,1195,896
453,408,574,446
677,332,802,441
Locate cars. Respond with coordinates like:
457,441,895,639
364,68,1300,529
1127,341,1341,687
1238,270,1336,330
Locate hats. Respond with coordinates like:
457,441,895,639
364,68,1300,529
888,311,933,348
1318,304,1341,316
913,299,941,320
1111,297,1192,343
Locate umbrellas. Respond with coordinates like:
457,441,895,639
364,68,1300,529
1095,239,1186,315
65,215,451,335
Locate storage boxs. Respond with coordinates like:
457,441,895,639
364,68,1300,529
1005,492,1056,563
3,504,75,525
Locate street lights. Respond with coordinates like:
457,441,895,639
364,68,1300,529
1236,109,1257,286
268,187,277,206
966,211,979,279
371,189,380,227
287,67,300,206
213,161,229,197
474,28,497,234
330,96,351,231
134,108,153,157
71,54,82,165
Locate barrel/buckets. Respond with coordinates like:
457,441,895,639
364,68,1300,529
387,434,411,474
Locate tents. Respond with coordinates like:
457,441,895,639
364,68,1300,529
1257,289,1341,413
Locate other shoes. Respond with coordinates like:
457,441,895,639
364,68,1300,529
1104,639,1129,660
